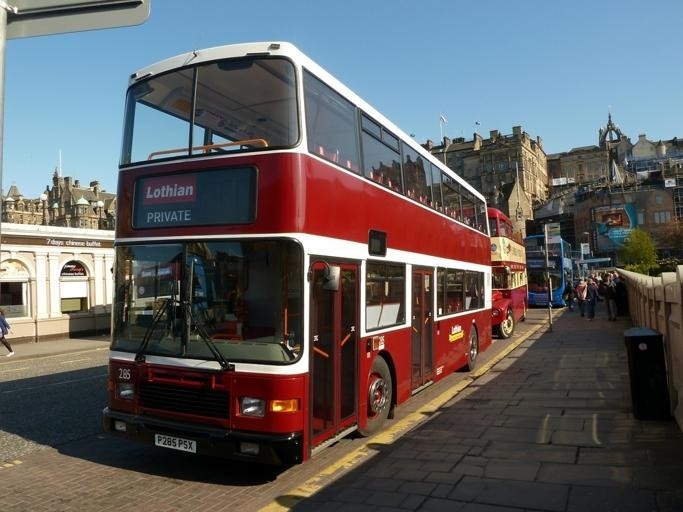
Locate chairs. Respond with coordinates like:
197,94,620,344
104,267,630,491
309,143,488,234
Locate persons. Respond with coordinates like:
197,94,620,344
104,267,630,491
0,308,14,359
567,270,623,320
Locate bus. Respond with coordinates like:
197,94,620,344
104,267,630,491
100,37,493,465
434,205,588,339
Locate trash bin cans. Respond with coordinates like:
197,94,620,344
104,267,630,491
624,328,671,421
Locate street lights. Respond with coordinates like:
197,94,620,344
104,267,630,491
40,193,47,226
95,199,104,229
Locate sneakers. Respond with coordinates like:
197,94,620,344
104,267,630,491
7,352,14,356
